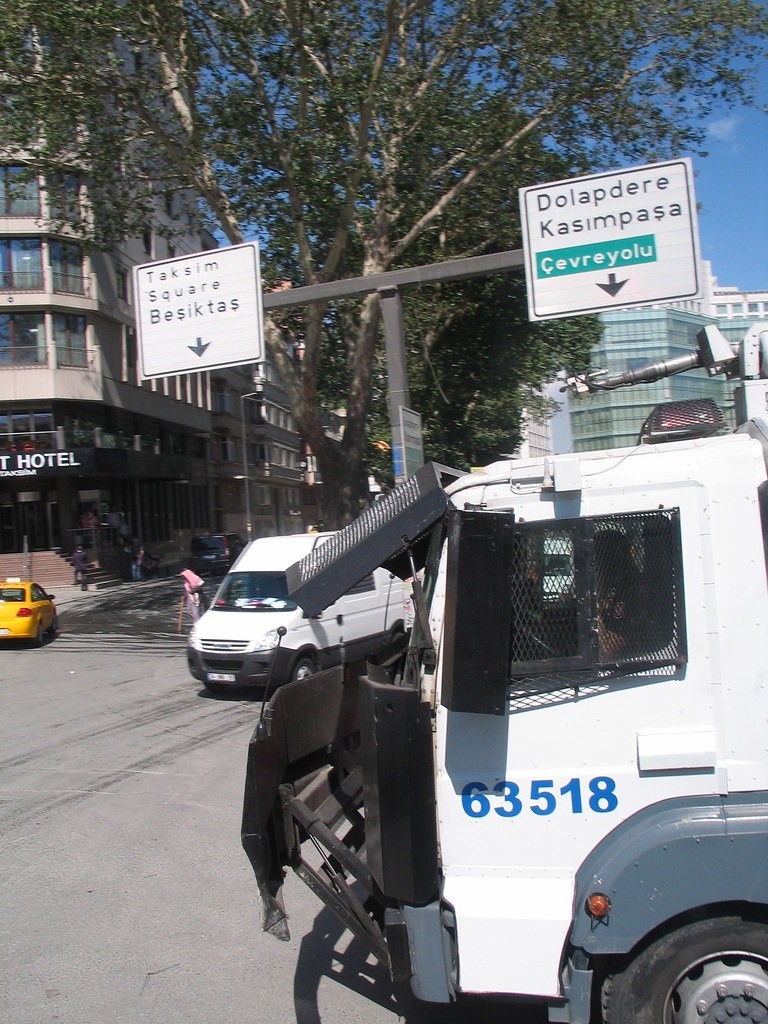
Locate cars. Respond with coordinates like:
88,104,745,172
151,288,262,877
191,533,247,576
0,582,57,646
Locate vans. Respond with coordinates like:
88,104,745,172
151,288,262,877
187,530,403,692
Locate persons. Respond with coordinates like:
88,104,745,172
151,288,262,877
125,537,144,582
105,508,130,546
556,530,655,659
80,512,100,528
180,568,210,622
71,546,88,591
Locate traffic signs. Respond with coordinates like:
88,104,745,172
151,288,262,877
518,157,706,323
131,243,266,381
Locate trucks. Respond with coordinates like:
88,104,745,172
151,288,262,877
241,324,767,1024
